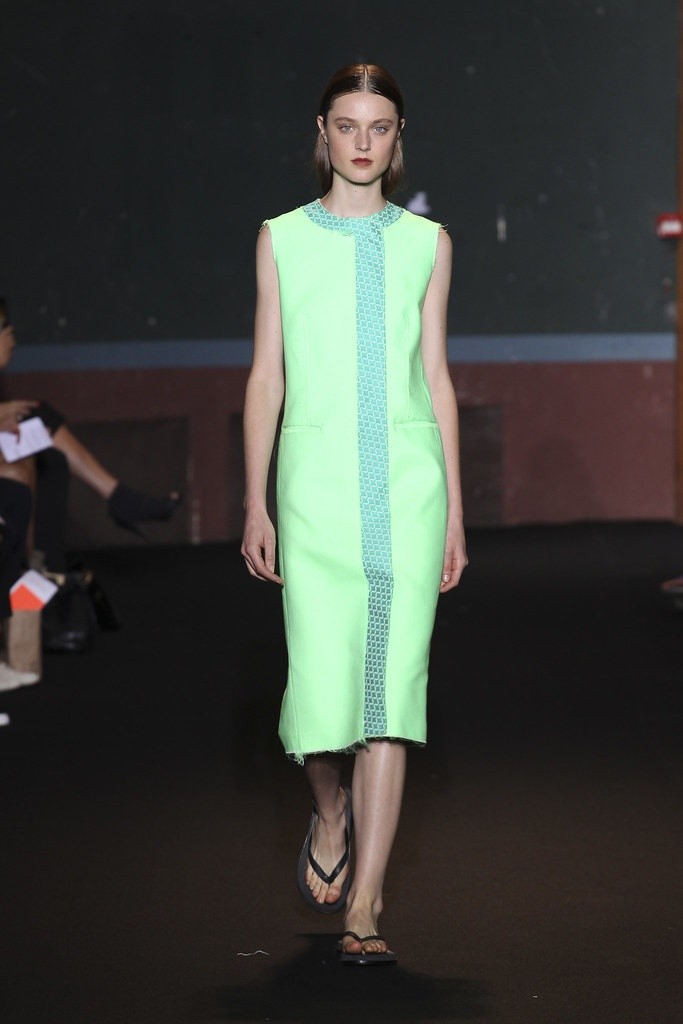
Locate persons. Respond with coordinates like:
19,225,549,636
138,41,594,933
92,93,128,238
0,298,180,692
240,62,468,964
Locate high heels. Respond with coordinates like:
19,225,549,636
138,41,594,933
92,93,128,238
106,479,184,542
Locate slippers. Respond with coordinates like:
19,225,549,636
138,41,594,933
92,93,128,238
297,787,356,911
339,920,398,965
